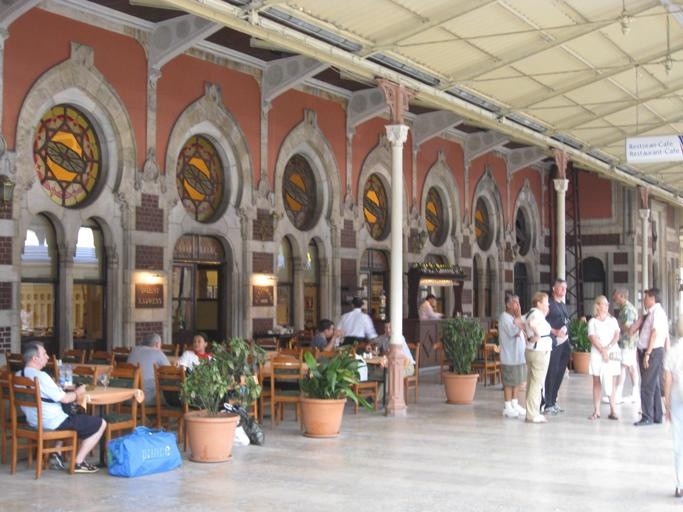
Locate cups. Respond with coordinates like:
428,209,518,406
456,312,462,318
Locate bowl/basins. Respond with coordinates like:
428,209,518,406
427,312,444,318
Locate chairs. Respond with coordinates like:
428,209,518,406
433,332,500,387
228,337,378,426
404,342,420,406
1,344,204,480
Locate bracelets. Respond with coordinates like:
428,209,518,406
73,390,78,399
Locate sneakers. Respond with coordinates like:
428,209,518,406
502,407,519,419
512,404,526,416
654,417,663,424
525,404,566,424
634,416,655,426
73,460,101,473
608,414,621,420
586,413,601,421
48,451,65,472
675,487,683,497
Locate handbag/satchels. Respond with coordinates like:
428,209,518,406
105,425,183,478
61,401,85,416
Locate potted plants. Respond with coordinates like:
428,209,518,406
569,317,591,375
177,335,262,463
441,317,484,405
298,348,373,438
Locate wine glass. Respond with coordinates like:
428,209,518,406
373,345,381,359
100,370,111,393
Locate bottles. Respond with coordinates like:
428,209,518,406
57,365,75,387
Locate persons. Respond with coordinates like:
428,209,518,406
493,277,672,425
417,292,446,321
333,296,378,354
125,331,171,429
358,320,416,411
662,337,683,500
176,331,216,372
11,339,108,474
308,319,343,371
